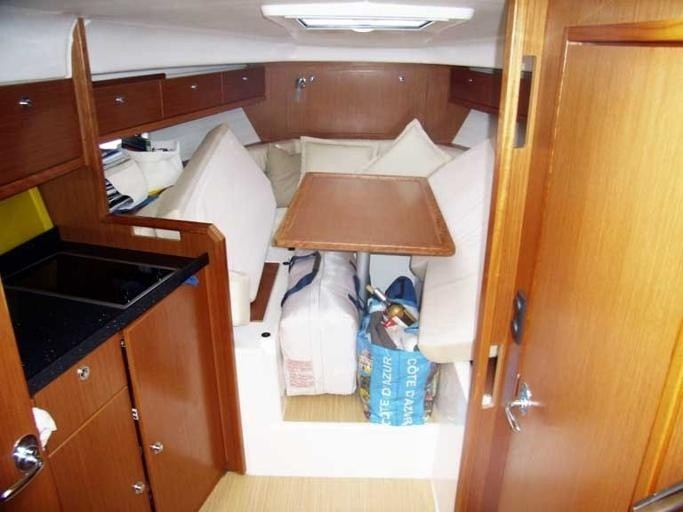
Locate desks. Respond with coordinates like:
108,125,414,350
271,172,456,317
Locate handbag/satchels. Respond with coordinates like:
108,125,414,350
281,246,357,397
357,276,442,426
119,138,184,193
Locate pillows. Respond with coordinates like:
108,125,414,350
298,116,453,188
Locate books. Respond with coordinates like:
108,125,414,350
100,133,159,214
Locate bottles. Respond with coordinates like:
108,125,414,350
366,285,417,327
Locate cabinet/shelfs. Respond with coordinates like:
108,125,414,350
449,66,531,124
31,253,153,511
0,65,266,201
116,255,226,512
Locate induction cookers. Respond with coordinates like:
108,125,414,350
0,250,180,312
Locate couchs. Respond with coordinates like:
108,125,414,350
126,136,472,422
